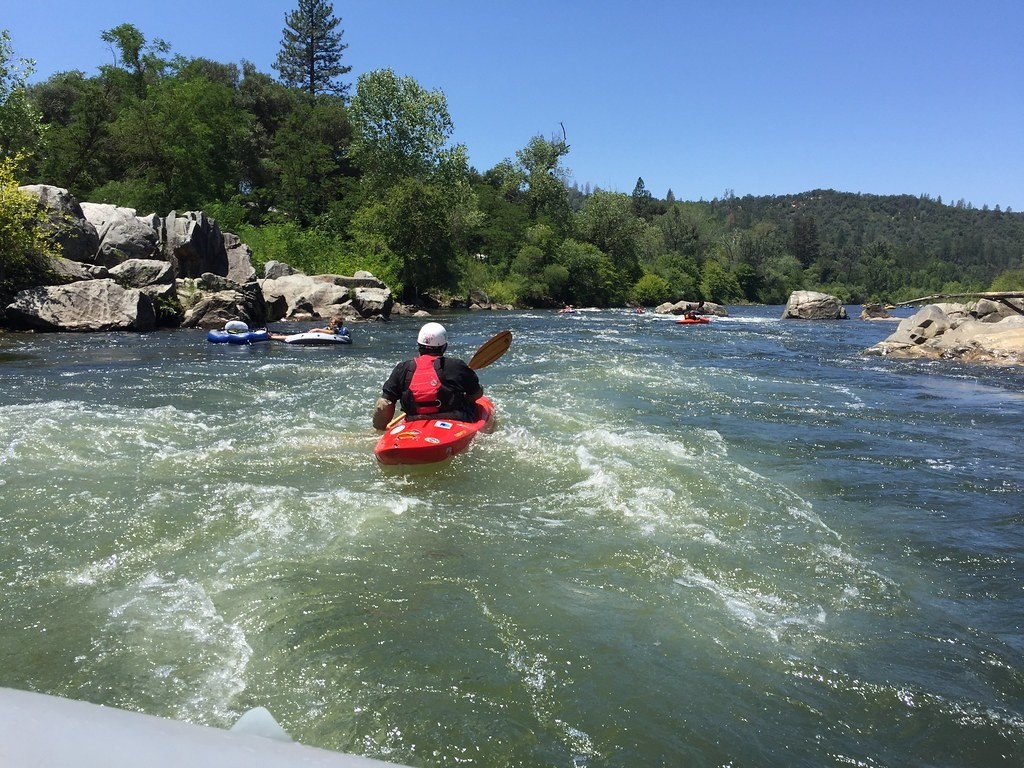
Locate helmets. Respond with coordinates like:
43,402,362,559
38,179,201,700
417,323,448,347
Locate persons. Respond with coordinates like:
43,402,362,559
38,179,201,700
560,305,575,312
684,304,700,320
373,322,483,431
268,316,343,338
636,306,642,313
229,305,252,331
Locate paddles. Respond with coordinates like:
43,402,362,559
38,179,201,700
559,304,574,318
695,300,705,311
384,329,515,427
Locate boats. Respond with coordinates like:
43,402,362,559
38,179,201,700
676,317,710,324
558,309,582,315
375,395,493,467
285,333,352,346
860,304,923,309
207,327,268,345
630,311,645,315
